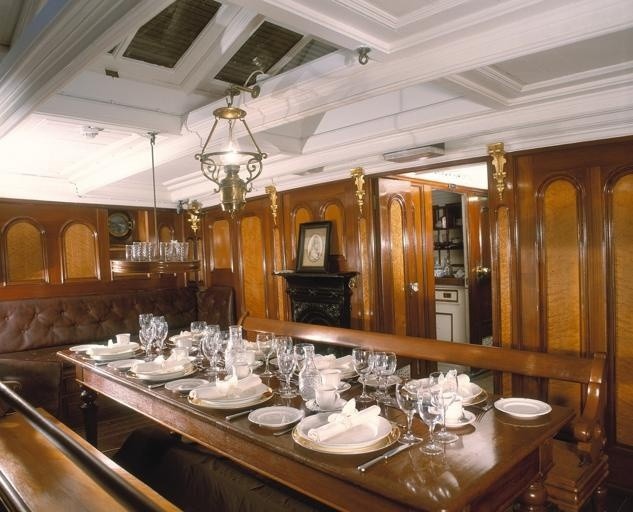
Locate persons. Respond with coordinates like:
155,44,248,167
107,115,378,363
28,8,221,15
306,235,322,262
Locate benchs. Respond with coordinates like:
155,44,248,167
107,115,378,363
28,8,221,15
237,312,608,512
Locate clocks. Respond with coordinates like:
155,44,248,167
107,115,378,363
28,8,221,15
109,210,134,239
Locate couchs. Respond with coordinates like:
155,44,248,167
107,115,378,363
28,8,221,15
0,286,234,421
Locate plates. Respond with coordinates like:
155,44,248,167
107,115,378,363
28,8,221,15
292,357,360,382
69,344,104,352
190,383,268,405
164,378,210,393
292,422,400,454
494,413,552,427
248,406,304,428
357,374,403,387
494,397,553,418
90,343,139,359
298,412,392,447
83,349,146,361
107,359,144,372
130,363,185,375
399,383,487,407
125,363,198,380
187,388,277,409
330,381,351,392
252,360,263,370
268,357,288,366
406,377,483,403
447,409,476,428
168,335,191,346
305,398,347,411
170,347,197,354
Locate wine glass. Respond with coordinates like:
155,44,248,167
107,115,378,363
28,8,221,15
272,336,293,383
396,379,423,444
257,332,275,377
417,388,445,455
156,321,168,352
375,352,397,404
277,348,300,400
429,372,459,443
352,348,374,402
191,322,230,378
367,355,388,402
294,343,313,373
138,329,149,358
139,313,154,327
142,322,156,361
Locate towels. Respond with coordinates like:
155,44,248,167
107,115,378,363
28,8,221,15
311,354,353,370
88,342,137,354
308,405,380,441
425,373,469,396
189,375,261,396
130,359,181,372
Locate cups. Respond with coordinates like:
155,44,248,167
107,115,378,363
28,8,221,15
159,242,167,258
320,369,342,388
175,242,185,259
132,242,141,261
246,350,255,364
125,245,133,260
147,242,152,260
316,391,341,410
163,244,173,261
177,336,192,350
170,240,177,258
141,242,147,260
116,333,131,346
152,316,164,321
233,361,253,379
216,375,237,392
184,242,188,258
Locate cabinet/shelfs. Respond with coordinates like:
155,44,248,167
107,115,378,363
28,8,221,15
433,203,466,342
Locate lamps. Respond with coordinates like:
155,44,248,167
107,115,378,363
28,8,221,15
194,84,268,219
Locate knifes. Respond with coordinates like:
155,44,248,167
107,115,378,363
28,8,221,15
357,442,413,470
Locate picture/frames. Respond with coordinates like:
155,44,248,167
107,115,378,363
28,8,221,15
296,222,331,273
